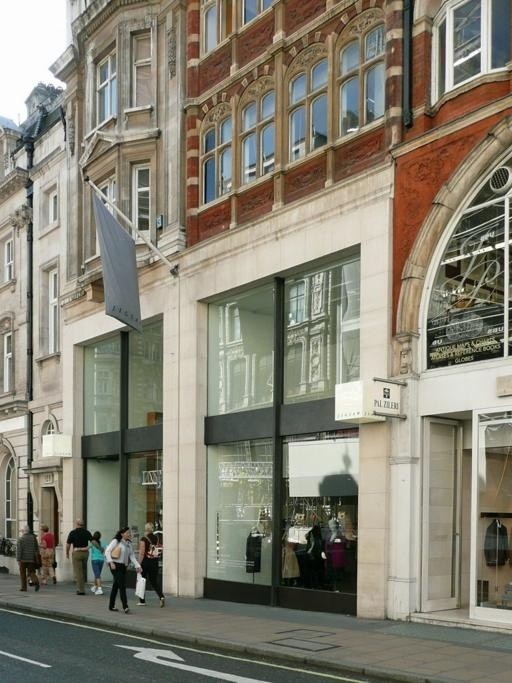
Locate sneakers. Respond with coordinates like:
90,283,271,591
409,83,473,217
34,584,40,591
91,585,104,595
76,589,85,596
159,597,166,607
52,576,57,584
135,602,147,606
124,607,130,613
20,588,27,592
109,607,119,612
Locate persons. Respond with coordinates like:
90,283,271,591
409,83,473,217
104,525,144,614
136,521,166,609
17,523,40,592
37,525,58,584
72,530,105,595
280,518,349,594
65,517,104,594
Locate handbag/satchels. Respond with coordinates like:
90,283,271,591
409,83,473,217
134,571,148,600
103,542,122,559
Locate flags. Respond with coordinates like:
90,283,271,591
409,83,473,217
91,189,141,331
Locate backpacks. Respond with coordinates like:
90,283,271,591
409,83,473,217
146,544,160,559
